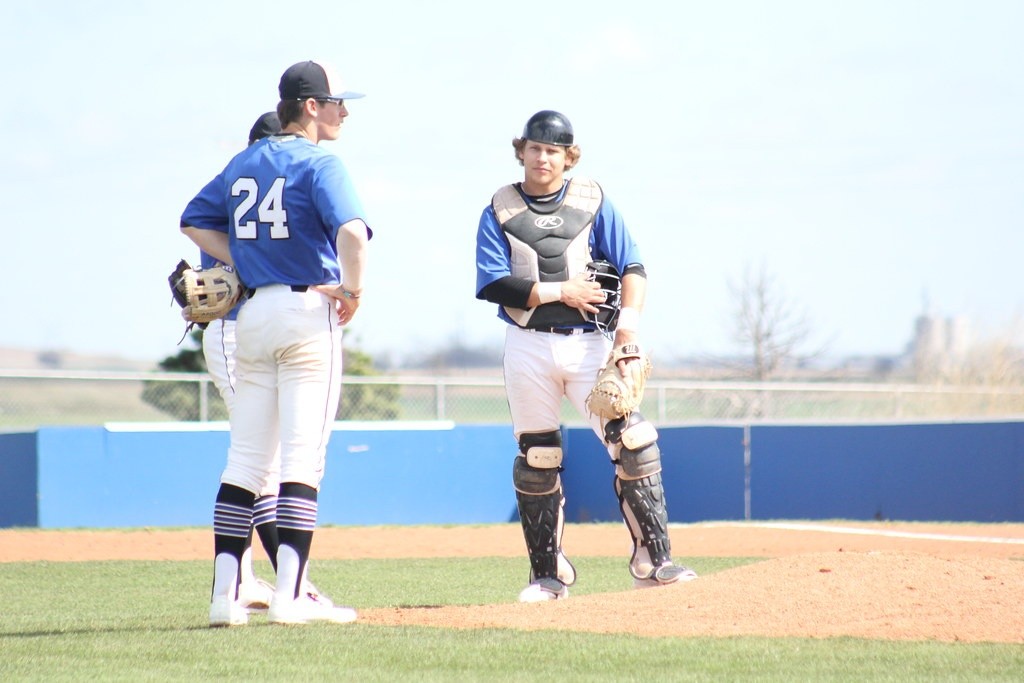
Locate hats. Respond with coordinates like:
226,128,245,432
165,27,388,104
249,112,282,139
278,60,367,100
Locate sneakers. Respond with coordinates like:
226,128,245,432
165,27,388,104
306,583,334,606
520,583,569,603
246,582,275,611
269,596,358,625
635,567,697,589
209,603,248,626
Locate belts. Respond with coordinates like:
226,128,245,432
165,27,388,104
248,284,310,299
535,326,595,337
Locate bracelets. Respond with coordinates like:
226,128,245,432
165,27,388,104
538,281,561,305
616,307,640,333
340,285,360,300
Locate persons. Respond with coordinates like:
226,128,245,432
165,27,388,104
178,59,374,625
473,110,696,602
202,112,282,608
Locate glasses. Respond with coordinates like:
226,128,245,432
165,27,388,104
297,97,343,106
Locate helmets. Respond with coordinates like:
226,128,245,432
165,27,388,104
521,111,574,146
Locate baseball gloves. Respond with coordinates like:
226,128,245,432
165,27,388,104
587,345,655,421
183,264,241,323
